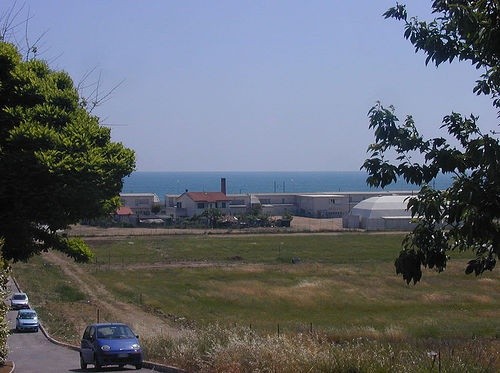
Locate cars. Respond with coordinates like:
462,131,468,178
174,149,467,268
16,309,40,332
79,322,144,370
9,293,29,309
